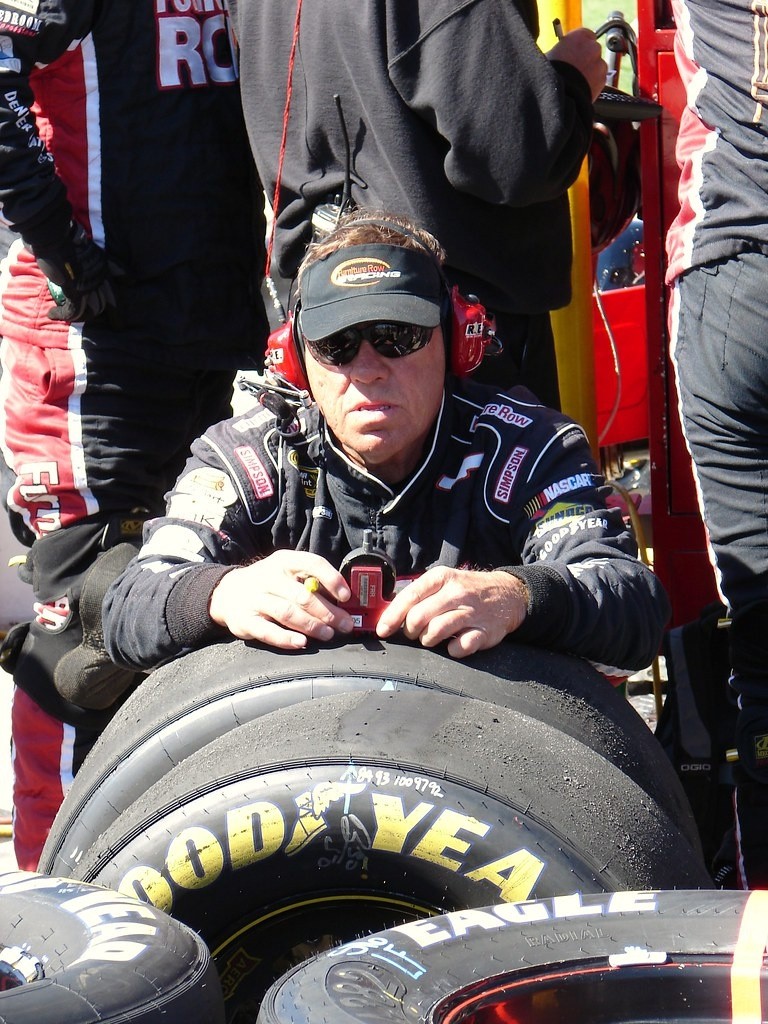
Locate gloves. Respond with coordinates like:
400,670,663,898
22,219,128,330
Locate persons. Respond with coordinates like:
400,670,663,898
665,0,768,891
225,0,610,415
100,209,673,689
0,0,271,874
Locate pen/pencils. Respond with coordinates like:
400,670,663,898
552,17,563,40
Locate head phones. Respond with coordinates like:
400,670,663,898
263,219,502,407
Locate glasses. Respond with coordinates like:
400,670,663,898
297,320,440,366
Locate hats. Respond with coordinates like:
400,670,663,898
300,243,441,341
